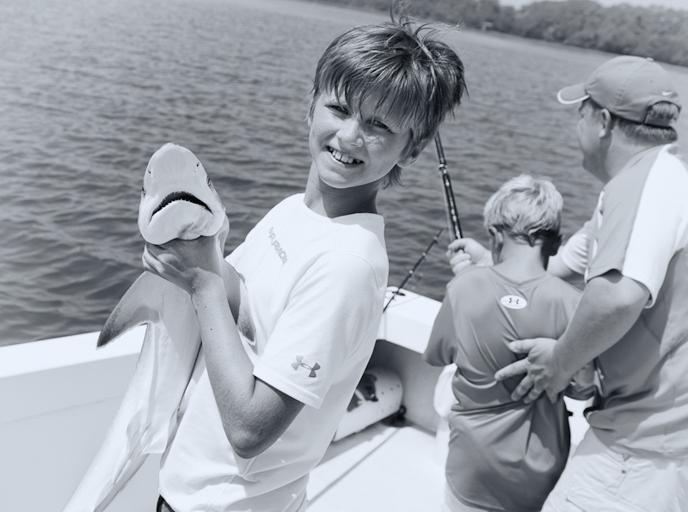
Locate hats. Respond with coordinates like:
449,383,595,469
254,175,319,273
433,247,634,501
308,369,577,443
557,56,681,127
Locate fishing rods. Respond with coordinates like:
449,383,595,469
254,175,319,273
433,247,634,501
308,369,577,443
383,227,445,313
399,0,464,253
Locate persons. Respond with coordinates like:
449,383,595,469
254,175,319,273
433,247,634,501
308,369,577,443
445,55,687,512
142,3,472,512
423,173,594,512
